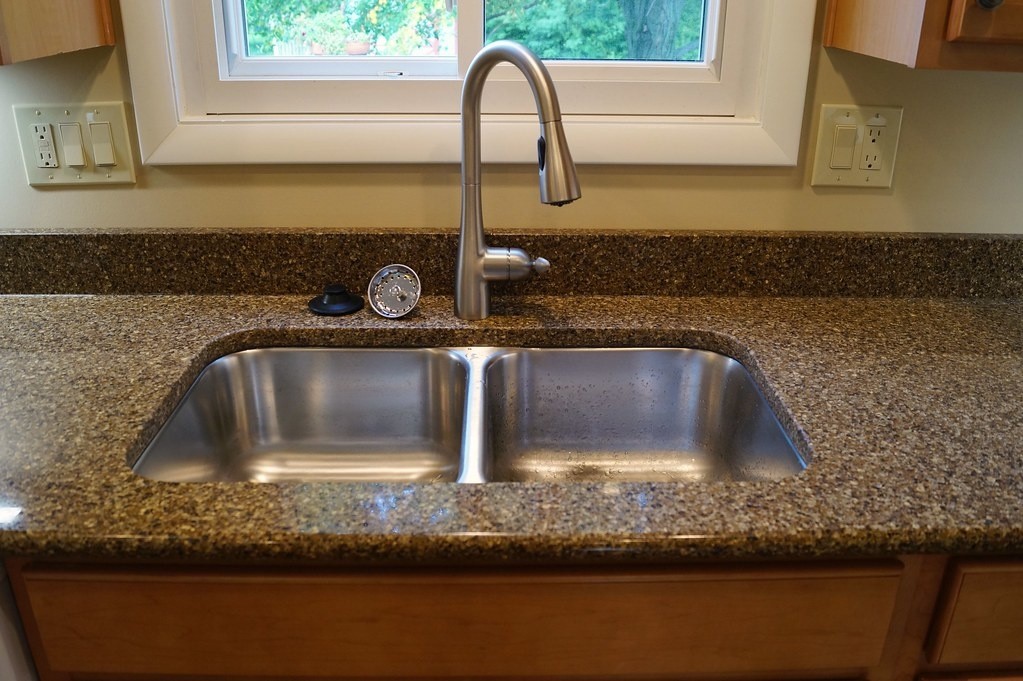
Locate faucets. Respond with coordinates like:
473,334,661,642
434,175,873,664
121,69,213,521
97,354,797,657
453,38,582,322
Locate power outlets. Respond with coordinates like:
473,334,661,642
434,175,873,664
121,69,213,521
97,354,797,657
13,100,137,188
810,103,904,189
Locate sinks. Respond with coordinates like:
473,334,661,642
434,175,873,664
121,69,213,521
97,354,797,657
124,326,473,486
472,327,816,483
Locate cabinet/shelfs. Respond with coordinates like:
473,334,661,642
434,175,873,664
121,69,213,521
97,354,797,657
822,0,1023,72
